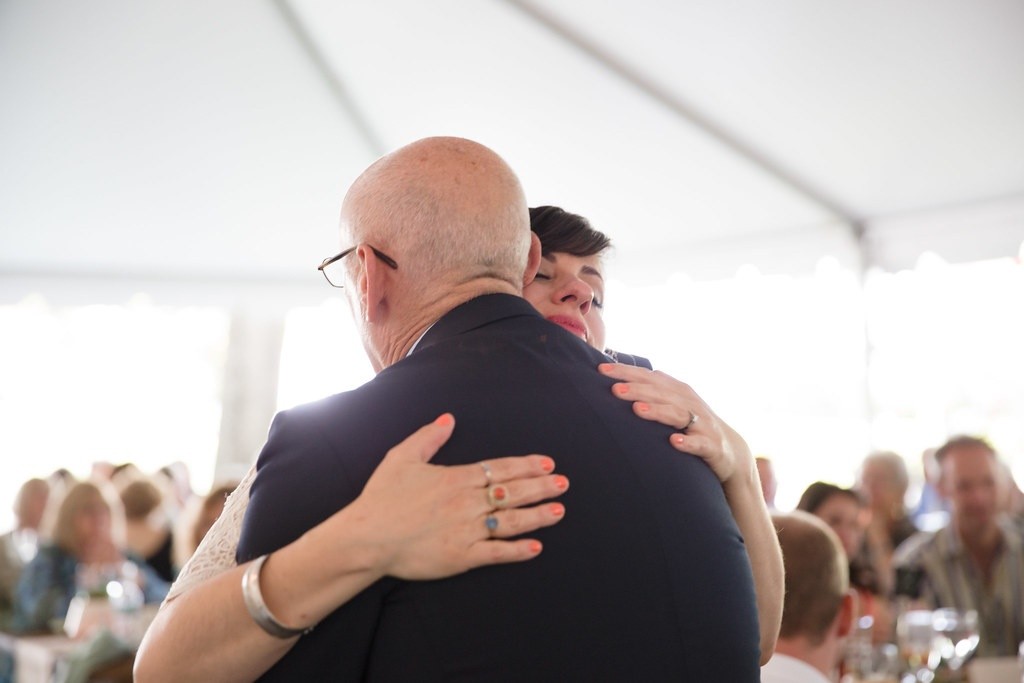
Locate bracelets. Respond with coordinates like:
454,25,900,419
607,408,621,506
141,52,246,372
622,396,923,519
242,554,309,640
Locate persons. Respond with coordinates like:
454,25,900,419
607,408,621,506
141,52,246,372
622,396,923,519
132,205,785,681
0,462,243,683
236,135,760,682
755,433,1024,683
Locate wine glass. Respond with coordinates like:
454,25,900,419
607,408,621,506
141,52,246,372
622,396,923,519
932,607,980,683
895,609,942,683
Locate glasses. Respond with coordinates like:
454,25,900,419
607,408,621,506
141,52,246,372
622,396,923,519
317,241,397,289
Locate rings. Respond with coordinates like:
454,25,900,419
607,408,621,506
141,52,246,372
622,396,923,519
682,410,699,431
488,484,509,509
484,513,498,539
480,461,493,485
